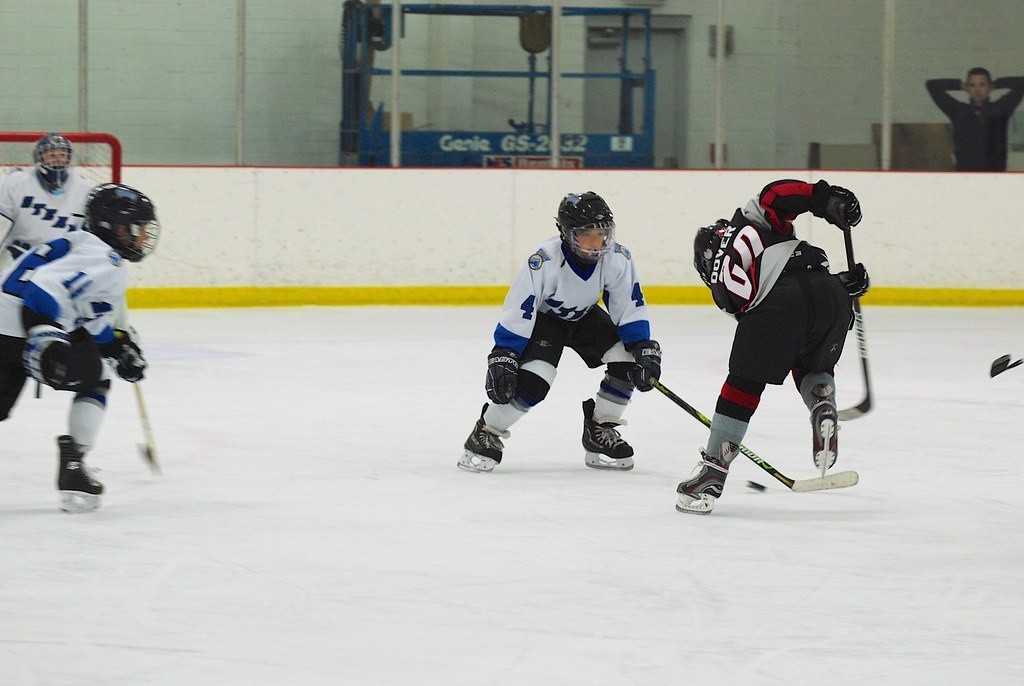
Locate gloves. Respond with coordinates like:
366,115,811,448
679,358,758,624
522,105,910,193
485,345,520,404
809,179,863,231
835,263,871,301
626,340,662,392
101,339,149,383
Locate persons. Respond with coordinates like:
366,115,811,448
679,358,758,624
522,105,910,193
676,179,872,515
0,136,160,513
924,68,1024,173
457,191,662,474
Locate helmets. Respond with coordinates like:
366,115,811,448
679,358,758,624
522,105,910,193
84,182,160,263
553,191,616,263
32,133,74,190
693,218,732,290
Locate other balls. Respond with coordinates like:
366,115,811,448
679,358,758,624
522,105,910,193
746,479,767,492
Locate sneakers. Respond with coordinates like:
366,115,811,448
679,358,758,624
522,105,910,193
457,402,511,474
809,395,842,470
673,445,731,515
55,434,105,514
582,397,635,471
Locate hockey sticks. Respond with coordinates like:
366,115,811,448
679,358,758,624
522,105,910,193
989,352,1024,378
135,380,157,465
650,378,860,492
835,203,874,423
